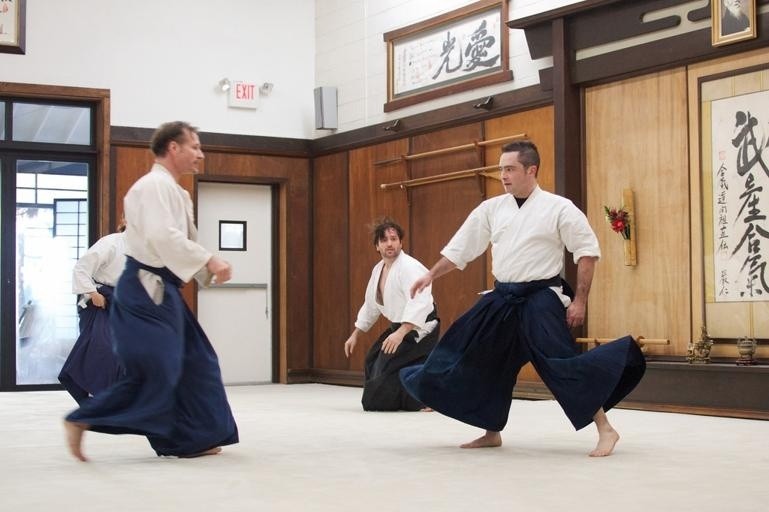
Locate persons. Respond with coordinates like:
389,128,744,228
64,121,241,461
399,142,646,456
344,223,441,414
59,215,131,405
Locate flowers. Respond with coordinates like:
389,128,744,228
603,205,631,240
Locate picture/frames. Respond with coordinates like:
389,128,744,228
383,0,513,113
0,0,26,54
711,0,758,47
